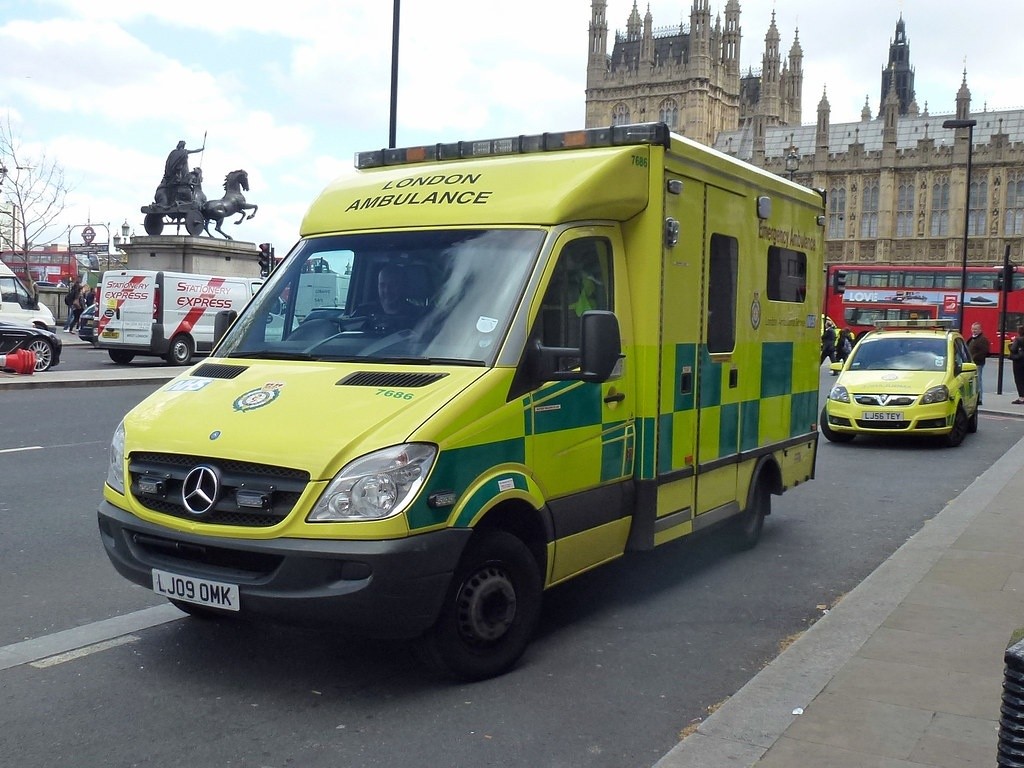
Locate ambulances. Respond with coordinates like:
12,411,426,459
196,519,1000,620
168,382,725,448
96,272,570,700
97,121,830,685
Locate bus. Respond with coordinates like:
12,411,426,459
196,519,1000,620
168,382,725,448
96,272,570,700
822,266,1024,357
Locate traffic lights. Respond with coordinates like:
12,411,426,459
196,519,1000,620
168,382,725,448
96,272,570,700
258,242,272,276
833,269,847,294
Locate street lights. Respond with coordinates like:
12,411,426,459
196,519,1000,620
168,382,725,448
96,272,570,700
943,119,978,333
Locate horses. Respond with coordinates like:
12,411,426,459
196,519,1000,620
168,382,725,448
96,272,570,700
155,166,258,241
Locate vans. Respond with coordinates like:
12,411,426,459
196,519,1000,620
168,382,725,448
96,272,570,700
97,269,266,367
0,259,57,334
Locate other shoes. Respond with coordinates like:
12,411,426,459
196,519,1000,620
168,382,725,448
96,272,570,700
69,330,76,334
831,373,838,376
1012,399,1024,404
64,329,69,333
829,370,834,374
978,401,982,405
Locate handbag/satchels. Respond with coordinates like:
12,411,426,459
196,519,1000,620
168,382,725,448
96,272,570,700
1008,339,1024,361
73,294,82,310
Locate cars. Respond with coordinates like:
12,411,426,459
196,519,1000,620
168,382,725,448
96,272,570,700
79,303,98,347
0,326,62,372
820,328,980,448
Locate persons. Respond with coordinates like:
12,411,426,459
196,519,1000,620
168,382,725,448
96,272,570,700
63,281,97,334
820,320,854,376
165,141,205,183
349,265,422,339
1008,325,1024,404
965,321,990,406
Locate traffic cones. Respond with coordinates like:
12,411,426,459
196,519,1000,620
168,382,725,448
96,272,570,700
0,348,35,375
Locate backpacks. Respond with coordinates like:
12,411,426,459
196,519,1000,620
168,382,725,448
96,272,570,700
840,337,852,354
64,291,75,306
